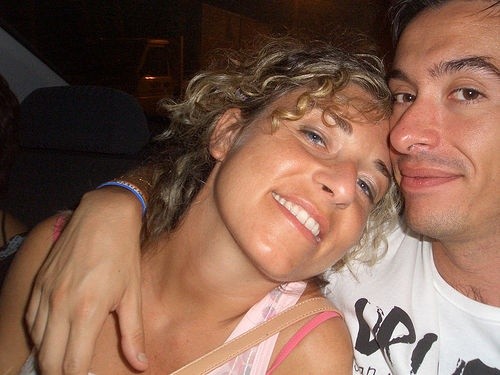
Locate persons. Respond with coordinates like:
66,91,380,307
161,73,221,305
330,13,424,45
1,26,404,374
21,1,500,374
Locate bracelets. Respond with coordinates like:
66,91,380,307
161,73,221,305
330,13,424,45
96,179,149,217
